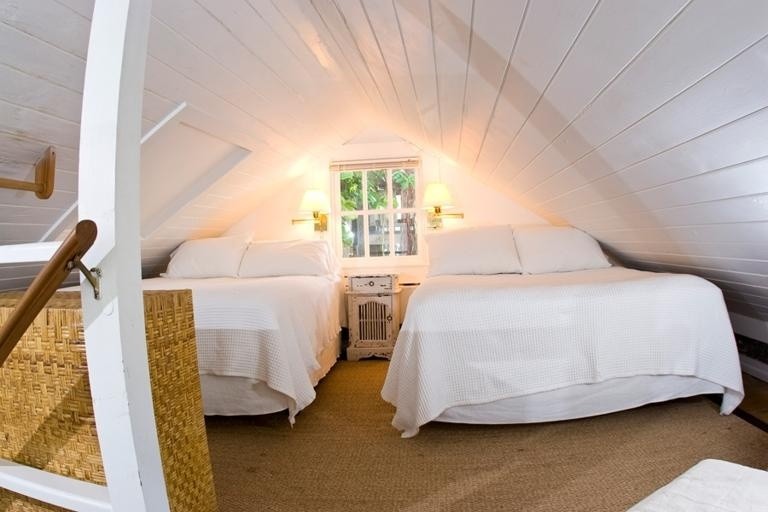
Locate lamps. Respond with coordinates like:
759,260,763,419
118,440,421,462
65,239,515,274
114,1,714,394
422,180,464,220
290,187,331,226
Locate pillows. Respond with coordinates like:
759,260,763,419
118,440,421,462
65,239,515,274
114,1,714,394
422,223,613,278
160,234,343,282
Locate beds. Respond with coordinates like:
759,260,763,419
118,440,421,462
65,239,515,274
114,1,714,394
402,263,726,433
55,273,345,419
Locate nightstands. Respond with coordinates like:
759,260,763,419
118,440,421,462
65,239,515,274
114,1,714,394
344,272,404,362
